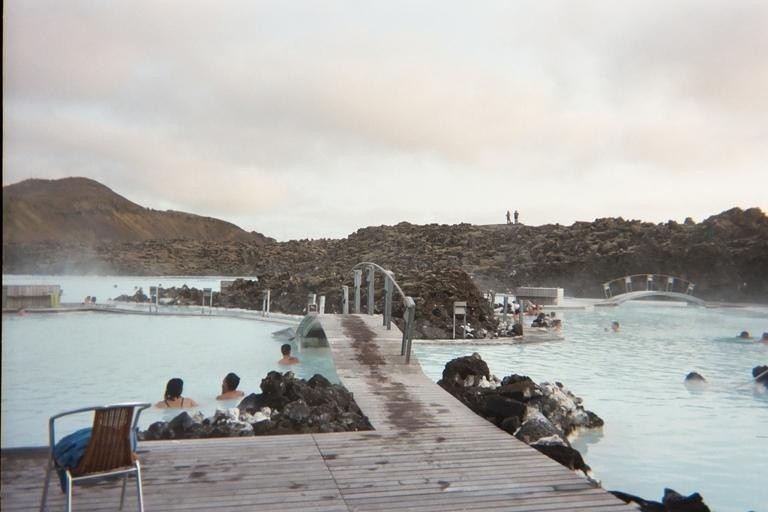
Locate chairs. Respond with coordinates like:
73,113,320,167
37,401,152,512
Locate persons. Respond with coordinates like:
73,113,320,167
278,344,299,365
216,372,245,400
737,331,751,338
506,211,512,224
749,366,768,392
84,296,96,303
685,373,706,383
493,299,564,334
758,332,768,344
156,378,196,409
514,211,519,223
603,321,619,333
